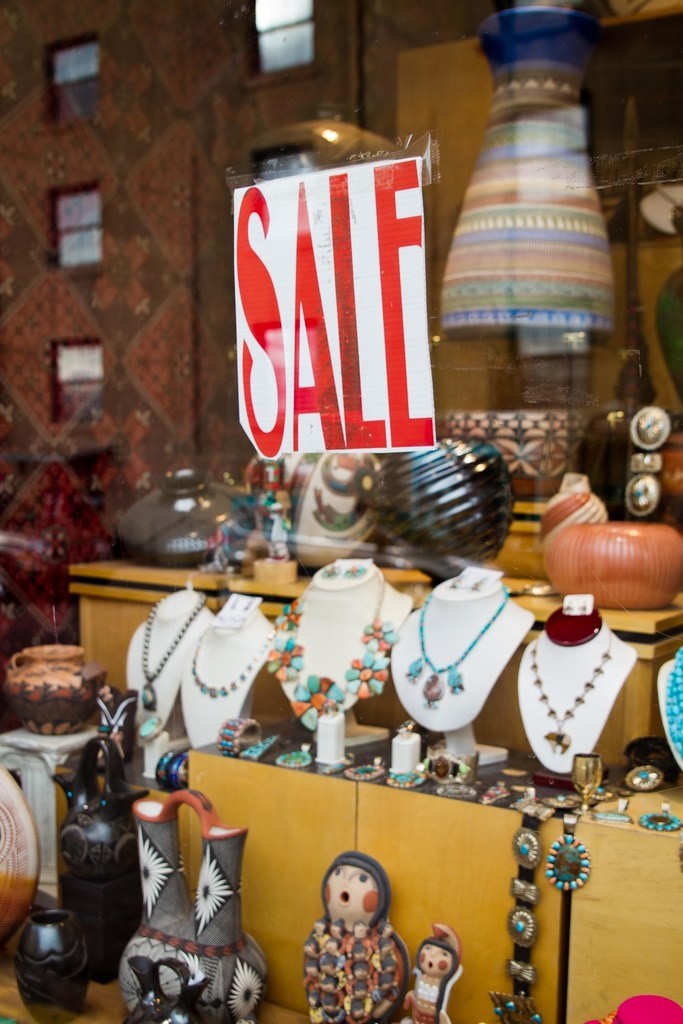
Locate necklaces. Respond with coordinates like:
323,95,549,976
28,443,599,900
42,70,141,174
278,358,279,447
137,574,683,763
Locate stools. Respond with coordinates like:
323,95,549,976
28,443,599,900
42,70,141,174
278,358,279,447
0,727,106,886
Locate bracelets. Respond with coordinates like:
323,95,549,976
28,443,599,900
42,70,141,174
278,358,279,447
155,719,426,788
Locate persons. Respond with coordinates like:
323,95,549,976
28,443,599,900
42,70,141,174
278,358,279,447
302,853,464,1024
